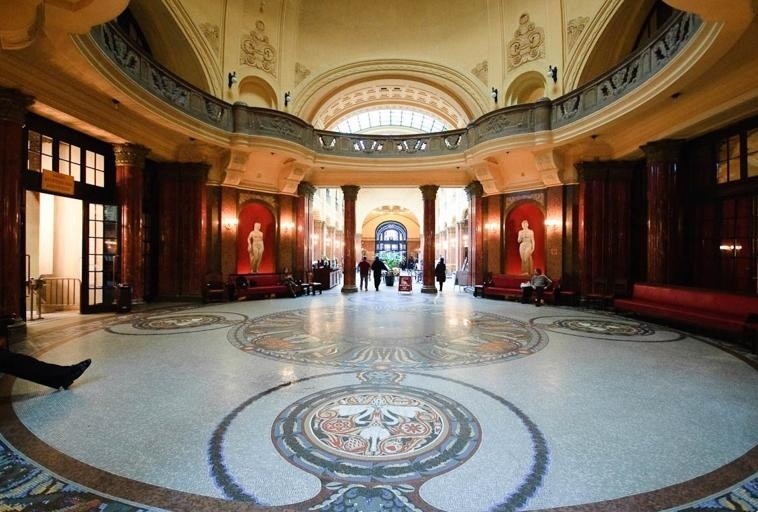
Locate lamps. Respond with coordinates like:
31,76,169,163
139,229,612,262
284,90,291,107
228,71,237,88
491,86,498,103
546,64,558,82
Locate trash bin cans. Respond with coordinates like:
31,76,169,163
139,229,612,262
117,284,132,314
385,272,395,286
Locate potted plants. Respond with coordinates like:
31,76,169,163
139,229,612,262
381,258,396,286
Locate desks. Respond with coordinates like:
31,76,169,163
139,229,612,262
301,282,323,295
559,289,576,307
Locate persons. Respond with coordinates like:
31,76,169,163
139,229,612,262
517,219,535,277
400,256,423,283
372,256,389,292
246,222,265,273
278,266,299,298
435,257,446,292
357,256,371,291
0,310,93,390
520,268,553,307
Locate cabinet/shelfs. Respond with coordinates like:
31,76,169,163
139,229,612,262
313,269,339,289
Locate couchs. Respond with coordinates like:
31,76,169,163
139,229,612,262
229,272,289,301
613,281,758,340
484,274,561,306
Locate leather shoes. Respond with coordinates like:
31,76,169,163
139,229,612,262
61,357,92,389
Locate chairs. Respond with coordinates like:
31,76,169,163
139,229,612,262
276,271,305,297
474,272,492,298
200,270,227,304
581,278,629,312
